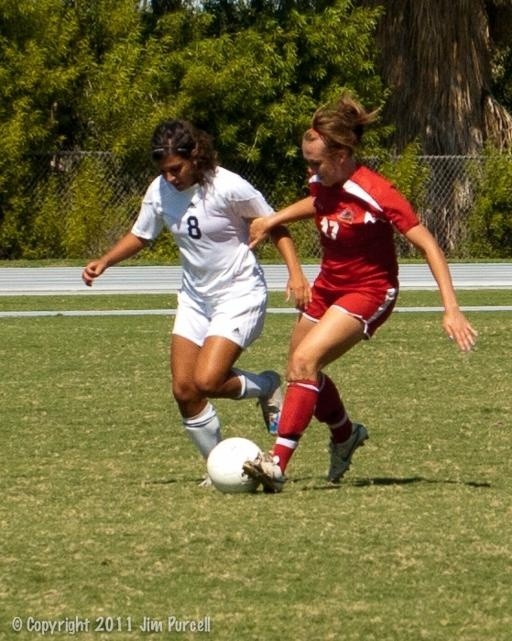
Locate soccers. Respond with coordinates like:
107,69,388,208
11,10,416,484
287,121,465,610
206,436,262,493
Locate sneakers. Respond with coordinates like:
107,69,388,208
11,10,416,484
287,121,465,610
257,370,285,436
241,450,287,493
325,423,369,484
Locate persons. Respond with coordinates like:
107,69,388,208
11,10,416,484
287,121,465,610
242,98,478,493
82,119,313,490
